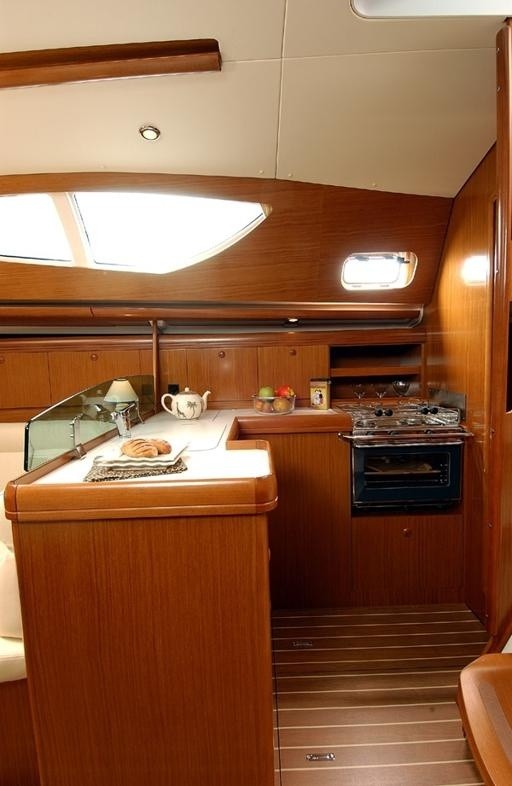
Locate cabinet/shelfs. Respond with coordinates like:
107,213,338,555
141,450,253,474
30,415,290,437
349,516,465,606
329,341,426,402
186,344,332,401
240,430,353,608
1,349,142,417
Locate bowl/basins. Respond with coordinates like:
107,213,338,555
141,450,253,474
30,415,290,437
252,394,295,415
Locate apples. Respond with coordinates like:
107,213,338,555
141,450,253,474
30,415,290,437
274,385,293,399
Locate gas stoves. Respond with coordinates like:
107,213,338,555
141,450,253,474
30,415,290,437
337,402,461,429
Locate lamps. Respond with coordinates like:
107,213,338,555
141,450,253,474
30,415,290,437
104,375,142,422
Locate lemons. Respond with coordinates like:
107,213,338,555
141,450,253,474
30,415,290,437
258,386,274,397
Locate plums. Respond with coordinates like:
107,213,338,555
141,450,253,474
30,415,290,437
263,402,273,412
255,400,264,410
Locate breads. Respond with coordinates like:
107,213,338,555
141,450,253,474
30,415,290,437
147,439,171,453
122,439,157,458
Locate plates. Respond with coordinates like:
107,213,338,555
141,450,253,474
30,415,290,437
98,447,189,467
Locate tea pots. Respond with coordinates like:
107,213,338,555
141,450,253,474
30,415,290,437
160,386,212,421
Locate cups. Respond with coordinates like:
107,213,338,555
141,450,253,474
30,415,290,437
351,381,366,399
391,377,410,396
372,379,390,397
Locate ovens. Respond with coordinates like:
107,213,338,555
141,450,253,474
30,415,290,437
343,432,467,512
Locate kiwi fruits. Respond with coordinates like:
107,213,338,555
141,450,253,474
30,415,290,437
273,399,291,412
